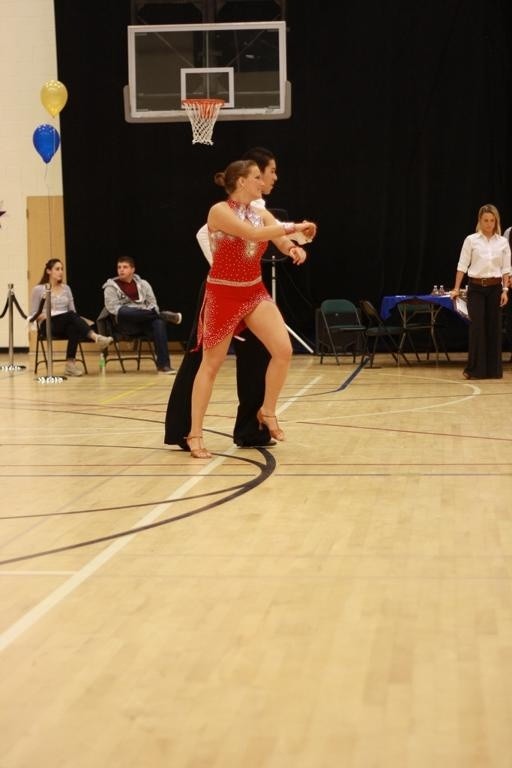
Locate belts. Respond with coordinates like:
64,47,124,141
471,277,501,287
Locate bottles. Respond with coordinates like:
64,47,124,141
438,285,444,296
432,285,438,296
99,353,106,375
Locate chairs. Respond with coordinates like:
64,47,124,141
35,314,88,374
102,307,159,374
320,299,450,368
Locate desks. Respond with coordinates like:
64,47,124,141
380,295,468,366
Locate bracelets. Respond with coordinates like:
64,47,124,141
289,246,296,252
283,222,295,235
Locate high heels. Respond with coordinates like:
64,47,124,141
257,409,284,441
185,431,211,459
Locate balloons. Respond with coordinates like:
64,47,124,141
32,123,60,164
40,79,69,119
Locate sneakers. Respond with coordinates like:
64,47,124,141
158,311,182,326
156,365,176,375
94,335,113,349
63,361,81,377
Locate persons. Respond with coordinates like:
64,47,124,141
32,258,113,376
183,160,319,460
164,147,314,452
101,256,182,375
450,205,512,380
